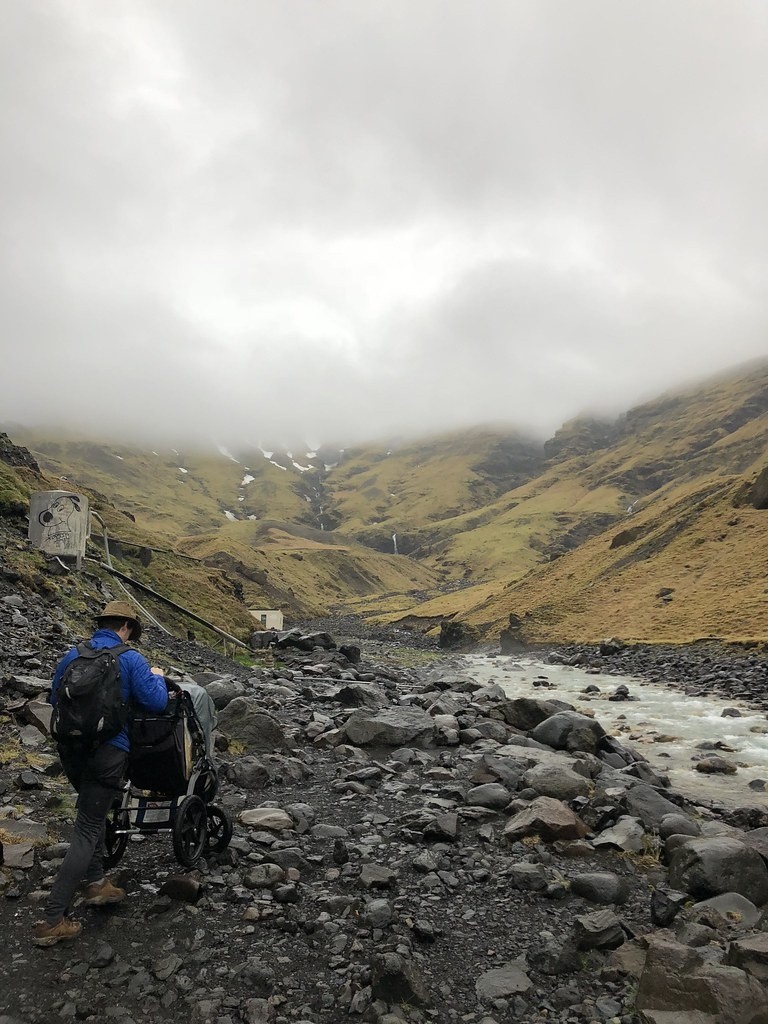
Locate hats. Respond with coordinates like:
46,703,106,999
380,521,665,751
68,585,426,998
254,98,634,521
91,600,142,640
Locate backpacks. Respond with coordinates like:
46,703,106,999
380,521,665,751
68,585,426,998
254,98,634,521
49,640,136,748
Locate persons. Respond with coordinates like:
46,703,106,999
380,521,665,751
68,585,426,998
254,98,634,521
33,601,169,947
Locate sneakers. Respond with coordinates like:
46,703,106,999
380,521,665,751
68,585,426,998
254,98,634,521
31,915,84,947
84,877,127,906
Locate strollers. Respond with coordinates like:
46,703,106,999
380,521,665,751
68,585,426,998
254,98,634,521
101,668,234,871
